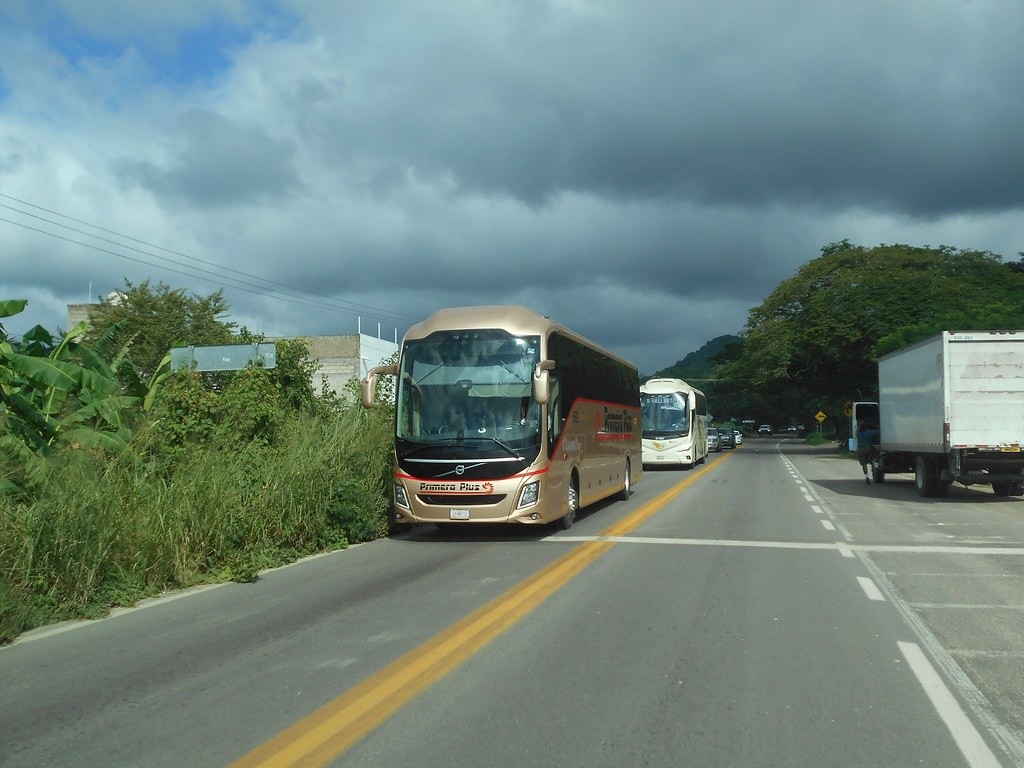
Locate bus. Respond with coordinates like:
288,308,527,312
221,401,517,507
359,303,642,529
638,377,709,469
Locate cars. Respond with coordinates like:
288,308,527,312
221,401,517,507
787,425,797,433
708,427,722,453
717,427,736,449
734,430,744,445
757,424,773,436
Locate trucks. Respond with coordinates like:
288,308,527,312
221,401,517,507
849,327,1024,495
